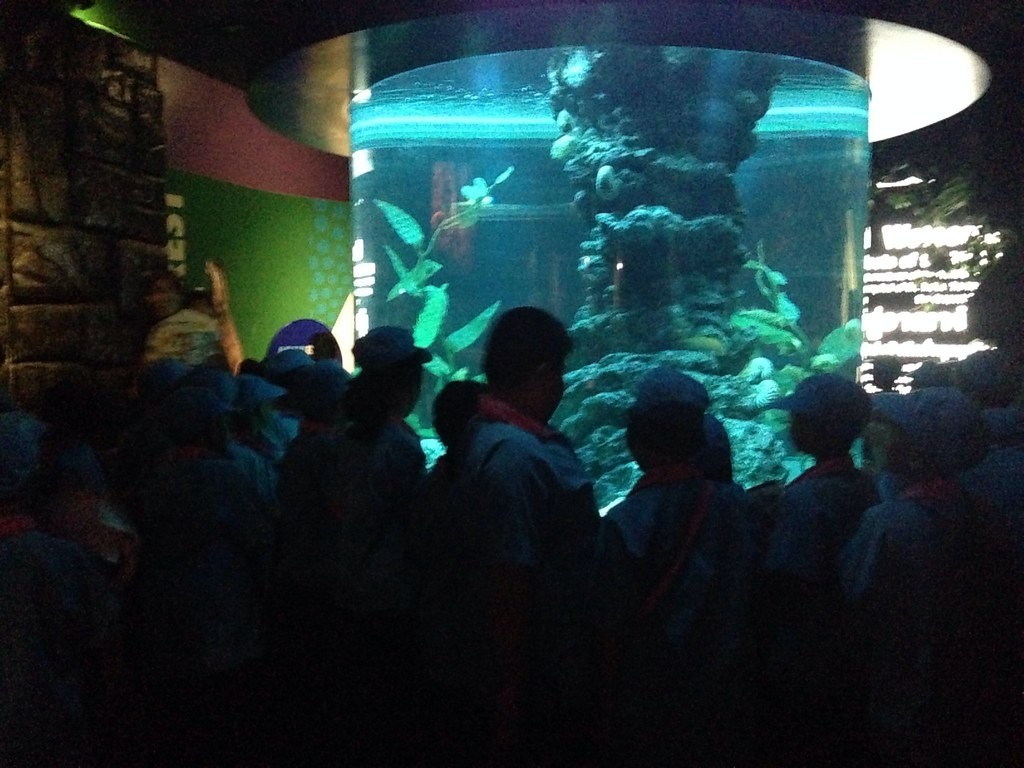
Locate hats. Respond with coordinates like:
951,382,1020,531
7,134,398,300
270,348,320,373
296,359,355,409
702,411,729,456
351,325,433,370
875,387,976,441
637,365,710,426
235,373,287,402
772,371,873,422
193,371,255,410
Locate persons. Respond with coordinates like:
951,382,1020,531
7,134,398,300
140,289,224,372
685,410,760,514
416,305,600,766
405,372,489,764
771,374,872,764
134,270,227,373
333,328,443,764
0,302,343,760
589,374,772,766
809,385,1013,768
